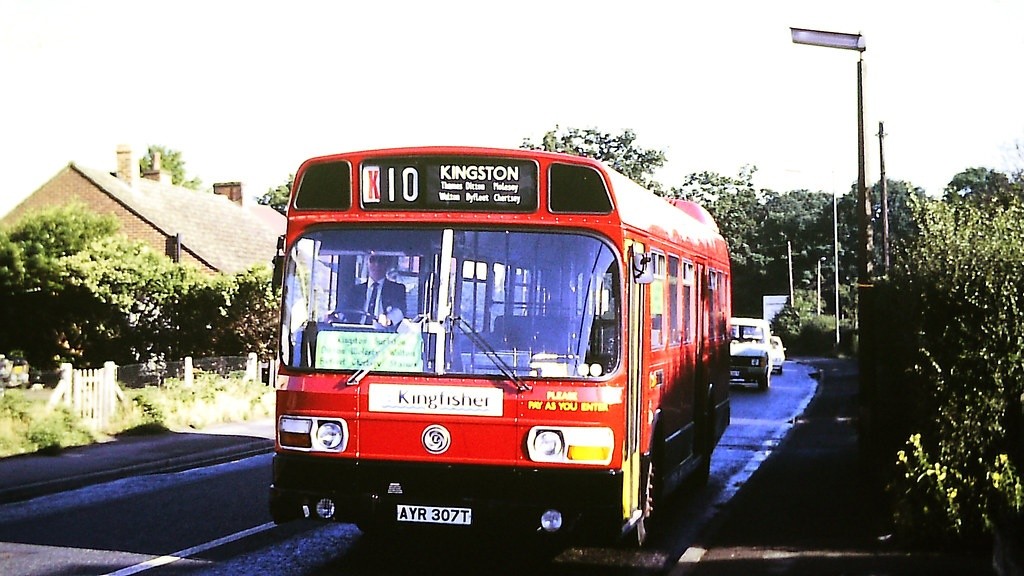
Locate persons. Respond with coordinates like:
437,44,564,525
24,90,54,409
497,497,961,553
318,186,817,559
325,256,408,328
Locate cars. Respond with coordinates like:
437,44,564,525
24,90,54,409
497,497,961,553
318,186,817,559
769,335,787,375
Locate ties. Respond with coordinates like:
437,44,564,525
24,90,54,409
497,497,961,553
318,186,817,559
365,282,379,325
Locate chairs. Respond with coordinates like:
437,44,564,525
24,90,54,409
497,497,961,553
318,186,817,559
494,313,618,365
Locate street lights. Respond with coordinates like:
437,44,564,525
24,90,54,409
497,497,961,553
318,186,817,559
788,25,878,448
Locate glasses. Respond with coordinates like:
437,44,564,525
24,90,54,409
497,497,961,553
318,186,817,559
367,256,388,264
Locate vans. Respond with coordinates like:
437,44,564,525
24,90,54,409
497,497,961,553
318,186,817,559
730,317,773,389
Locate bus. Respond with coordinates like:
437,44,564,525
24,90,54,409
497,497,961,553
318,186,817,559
267,143,734,549
